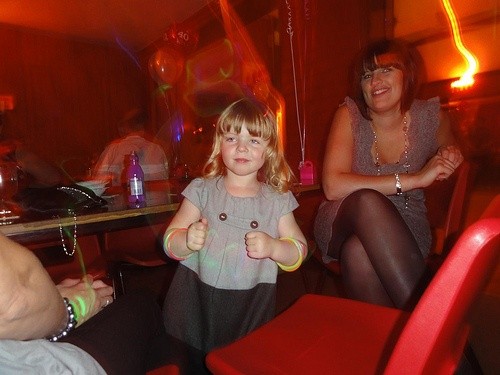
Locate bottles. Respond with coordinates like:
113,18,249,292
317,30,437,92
127,153,144,204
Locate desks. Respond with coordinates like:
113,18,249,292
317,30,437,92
0,178,323,258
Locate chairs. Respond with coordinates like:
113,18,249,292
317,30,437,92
304,157,473,295
204,211,500,375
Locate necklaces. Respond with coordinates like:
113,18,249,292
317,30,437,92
370,111,409,212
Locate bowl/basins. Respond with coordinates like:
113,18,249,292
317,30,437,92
75,180,110,197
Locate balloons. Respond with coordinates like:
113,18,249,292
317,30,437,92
155,46,183,85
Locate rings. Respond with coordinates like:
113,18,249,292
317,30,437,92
105,298,110,306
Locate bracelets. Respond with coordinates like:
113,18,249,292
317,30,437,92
276,236,307,272
47,298,76,342
163,228,193,261
394,174,403,195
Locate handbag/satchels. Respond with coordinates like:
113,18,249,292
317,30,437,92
16,185,108,215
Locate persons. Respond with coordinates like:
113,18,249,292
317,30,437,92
312,38,485,375
0,231,165,375
93,105,170,181
163,96,308,375
